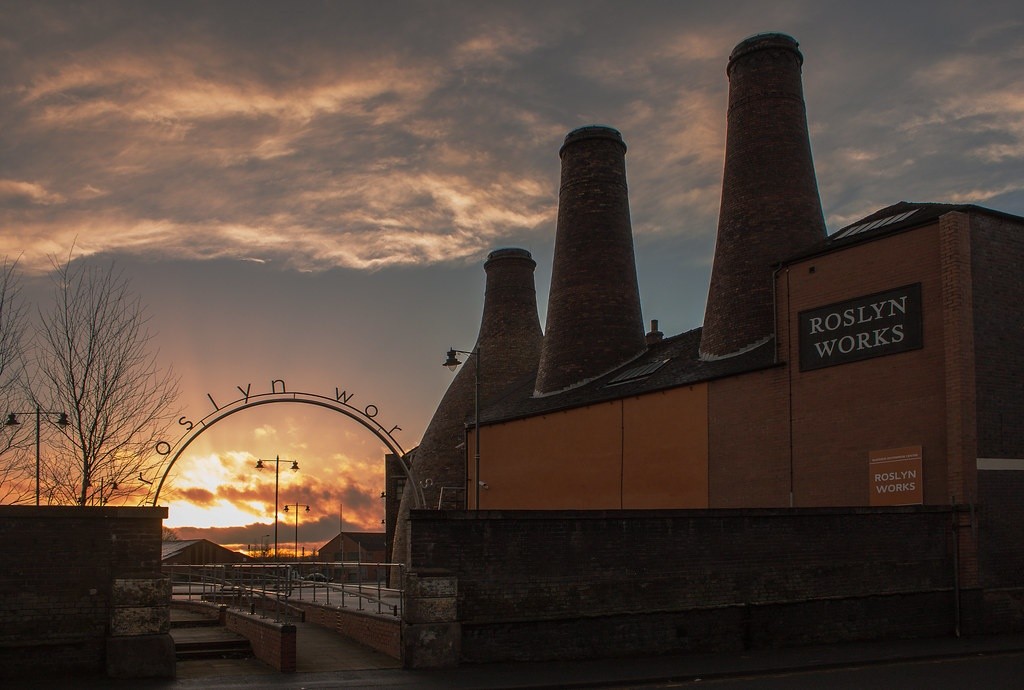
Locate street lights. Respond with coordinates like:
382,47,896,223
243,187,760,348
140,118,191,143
283,501,310,560
5,405,69,506
253,454,300,560
77,480,118,506
260,534,270,551
443,346,482,510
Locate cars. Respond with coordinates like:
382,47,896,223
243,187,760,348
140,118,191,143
306,573,327,582
298,574,303,579
